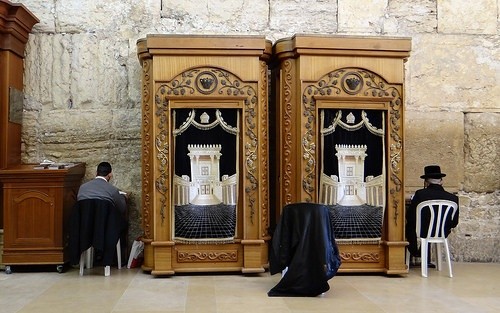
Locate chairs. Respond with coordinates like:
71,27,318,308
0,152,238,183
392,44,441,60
77,196,124,276
405,199,458,278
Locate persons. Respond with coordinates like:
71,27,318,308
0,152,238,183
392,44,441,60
403,164,460,256
77,159,128,215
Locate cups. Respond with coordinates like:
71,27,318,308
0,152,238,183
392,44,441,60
58,165,64,170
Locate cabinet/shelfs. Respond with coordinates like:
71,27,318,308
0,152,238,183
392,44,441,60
133,33,273,276
276,34,413,277
0,159,85,266
0,0,41,168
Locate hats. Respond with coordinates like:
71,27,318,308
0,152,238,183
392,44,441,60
419,165,447,181
